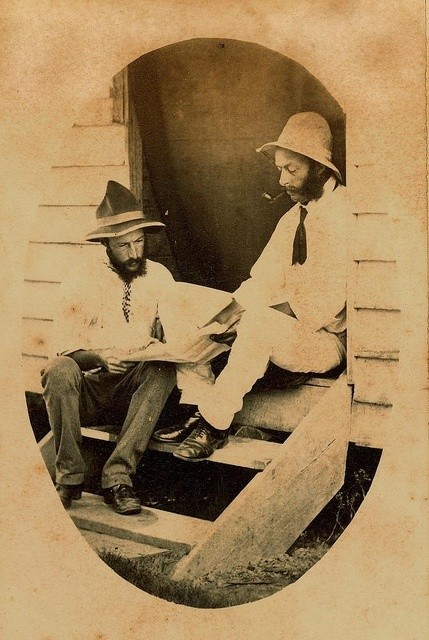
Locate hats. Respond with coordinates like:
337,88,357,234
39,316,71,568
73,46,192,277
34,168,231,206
256,110,343,185
83,179,166,243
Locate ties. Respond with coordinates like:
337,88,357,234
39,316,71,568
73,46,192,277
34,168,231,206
122,281,132,322
292,208,307,265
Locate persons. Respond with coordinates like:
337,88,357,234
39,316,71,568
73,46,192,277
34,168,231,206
38,181,239,515
153,110,349,461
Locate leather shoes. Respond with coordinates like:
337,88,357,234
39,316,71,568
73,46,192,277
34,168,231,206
56,484,83,507
104,485,141,515
152,411,200,443
173,422,229,462
